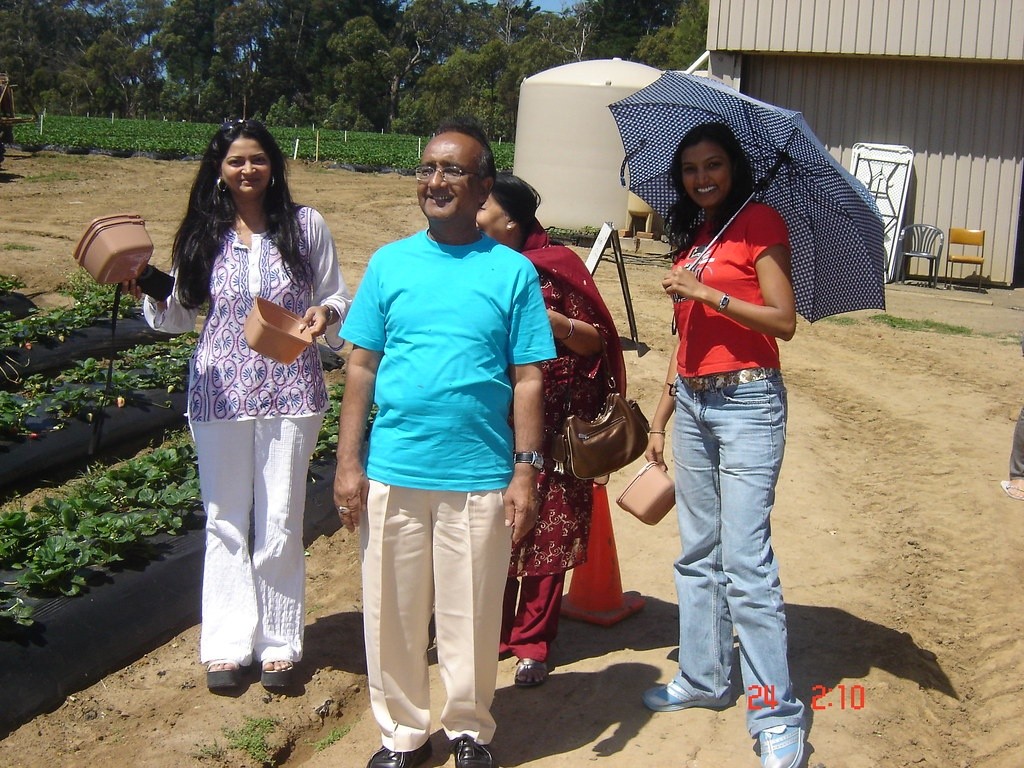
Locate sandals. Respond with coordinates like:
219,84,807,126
514,659,548,685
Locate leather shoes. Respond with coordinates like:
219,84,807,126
455,734,493,768
369,737,432,768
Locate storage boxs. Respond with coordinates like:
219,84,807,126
244,298,313,364
73,212,153,285
615,460,675,526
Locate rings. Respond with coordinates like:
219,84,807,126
338,505,347,513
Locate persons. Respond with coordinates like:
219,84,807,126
641,122,804,768
119,117,353,689
332,126,558,768
1000,407,1024,500
423,171,626,687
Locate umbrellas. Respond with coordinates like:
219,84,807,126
609,69,886,325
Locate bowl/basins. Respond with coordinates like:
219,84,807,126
244,298,312,365
616,461,676,526
72,213,153,285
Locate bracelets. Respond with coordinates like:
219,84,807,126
561,318,575,340
513,451,545,471
324,304,333,323
717,292,730,313
649,431,665,434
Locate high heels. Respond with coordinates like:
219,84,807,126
206,660,240,685
261,661,294,686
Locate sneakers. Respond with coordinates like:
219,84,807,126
641,681,731,711
758,714,805,768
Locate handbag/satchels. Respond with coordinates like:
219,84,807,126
551,390,651,481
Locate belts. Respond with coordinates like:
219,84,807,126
677,368,774,394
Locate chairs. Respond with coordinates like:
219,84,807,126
895,224,944,288
945,227,985,293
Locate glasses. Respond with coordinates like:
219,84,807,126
415,166,481,184
221,120,263,132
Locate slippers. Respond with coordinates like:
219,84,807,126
1000,481,1024,500
